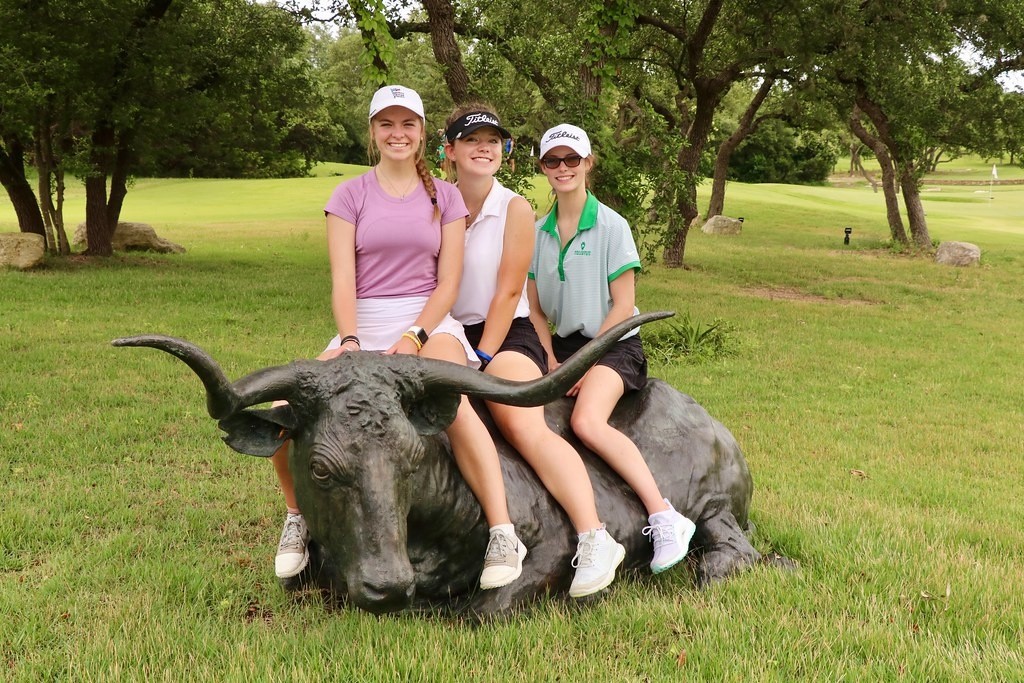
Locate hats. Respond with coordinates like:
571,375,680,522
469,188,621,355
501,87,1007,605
446,112,511,143
369,85,425,126
539,124,591,160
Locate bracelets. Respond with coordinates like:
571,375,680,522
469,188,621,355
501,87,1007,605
402,333,421,350
473,348,491,372
341,335,360,347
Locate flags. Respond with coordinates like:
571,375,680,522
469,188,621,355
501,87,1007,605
992,165,998,178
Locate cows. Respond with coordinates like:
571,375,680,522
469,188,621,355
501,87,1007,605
109,308,764,626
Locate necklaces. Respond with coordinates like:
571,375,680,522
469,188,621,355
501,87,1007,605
466,207,481,227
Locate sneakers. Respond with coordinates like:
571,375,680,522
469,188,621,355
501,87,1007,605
569,523,626,597
480,528,527,590
642,498,696,574
275,513,312,579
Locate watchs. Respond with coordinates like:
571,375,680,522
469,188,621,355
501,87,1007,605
408,326,429,345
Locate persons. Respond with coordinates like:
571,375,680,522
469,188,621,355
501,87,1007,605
444,103,626,598
527,122,696,574
274,85,527,589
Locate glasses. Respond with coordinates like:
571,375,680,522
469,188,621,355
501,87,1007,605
542,155,582,169
